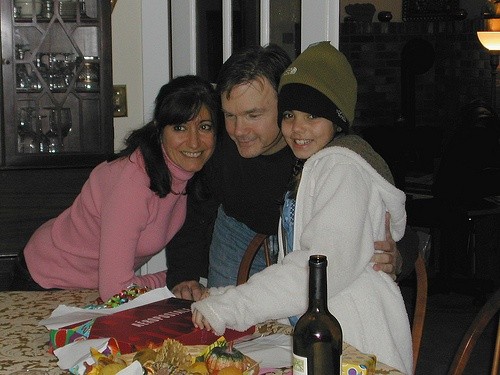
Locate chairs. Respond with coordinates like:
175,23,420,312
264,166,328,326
237,228,500,375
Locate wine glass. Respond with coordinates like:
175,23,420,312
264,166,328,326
16,106,72,153
14,42,75,92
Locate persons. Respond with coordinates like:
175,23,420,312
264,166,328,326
164,42,420,299
8,73,224,306
191,42,414,375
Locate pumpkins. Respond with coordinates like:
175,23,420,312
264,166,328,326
83,341,246,375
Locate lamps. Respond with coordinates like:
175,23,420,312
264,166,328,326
476,31,500,50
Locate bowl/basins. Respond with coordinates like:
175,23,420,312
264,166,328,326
14,0,43,19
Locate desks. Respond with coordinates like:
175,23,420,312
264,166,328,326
0,291,407,375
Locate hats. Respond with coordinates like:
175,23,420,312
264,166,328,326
278,41,357,133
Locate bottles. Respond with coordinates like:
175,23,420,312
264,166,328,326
75,55,102,93
292,255,343,375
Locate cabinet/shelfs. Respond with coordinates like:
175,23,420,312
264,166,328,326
0,0,114,294
